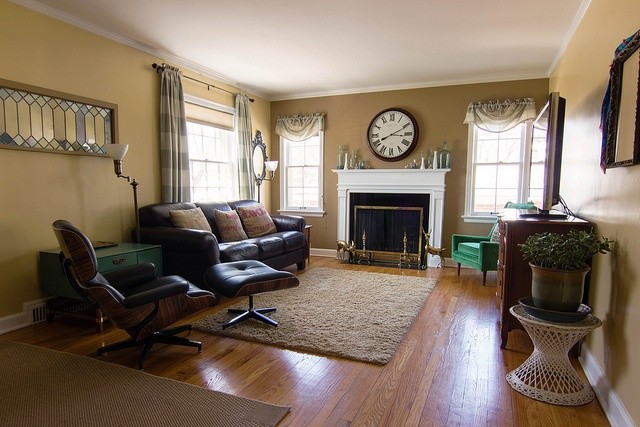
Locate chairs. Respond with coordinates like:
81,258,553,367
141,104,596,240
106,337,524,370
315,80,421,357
450,201,535,284
52,219,202,370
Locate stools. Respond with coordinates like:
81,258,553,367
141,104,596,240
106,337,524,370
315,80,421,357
204,259,300,329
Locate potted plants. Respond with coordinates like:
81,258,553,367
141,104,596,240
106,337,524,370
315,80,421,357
521,229,613,308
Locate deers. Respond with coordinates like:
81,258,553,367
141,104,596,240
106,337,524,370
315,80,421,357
422,227,447,268
336,240,355,262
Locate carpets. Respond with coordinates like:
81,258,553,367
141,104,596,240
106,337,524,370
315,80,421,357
1,341,292,427
190,265,437,366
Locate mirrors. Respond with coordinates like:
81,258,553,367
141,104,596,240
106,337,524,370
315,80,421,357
251,143,267,179
613,51,639,163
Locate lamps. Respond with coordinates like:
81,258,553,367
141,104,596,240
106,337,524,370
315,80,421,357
264,160,279,173
104,143,129,176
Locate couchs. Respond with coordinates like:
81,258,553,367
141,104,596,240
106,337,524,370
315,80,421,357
132,200,307,270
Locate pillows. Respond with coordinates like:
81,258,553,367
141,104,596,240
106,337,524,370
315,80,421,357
214,208,249,241
169,206,213,234
236,203,277,237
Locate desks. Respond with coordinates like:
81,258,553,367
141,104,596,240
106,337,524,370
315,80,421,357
302,224,314,264
507,306,602,407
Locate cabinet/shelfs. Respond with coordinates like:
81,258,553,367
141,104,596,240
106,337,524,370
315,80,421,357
38,242,161,333
494,208,591,334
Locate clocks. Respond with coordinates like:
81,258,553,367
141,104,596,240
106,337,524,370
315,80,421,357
366,107,420,161
